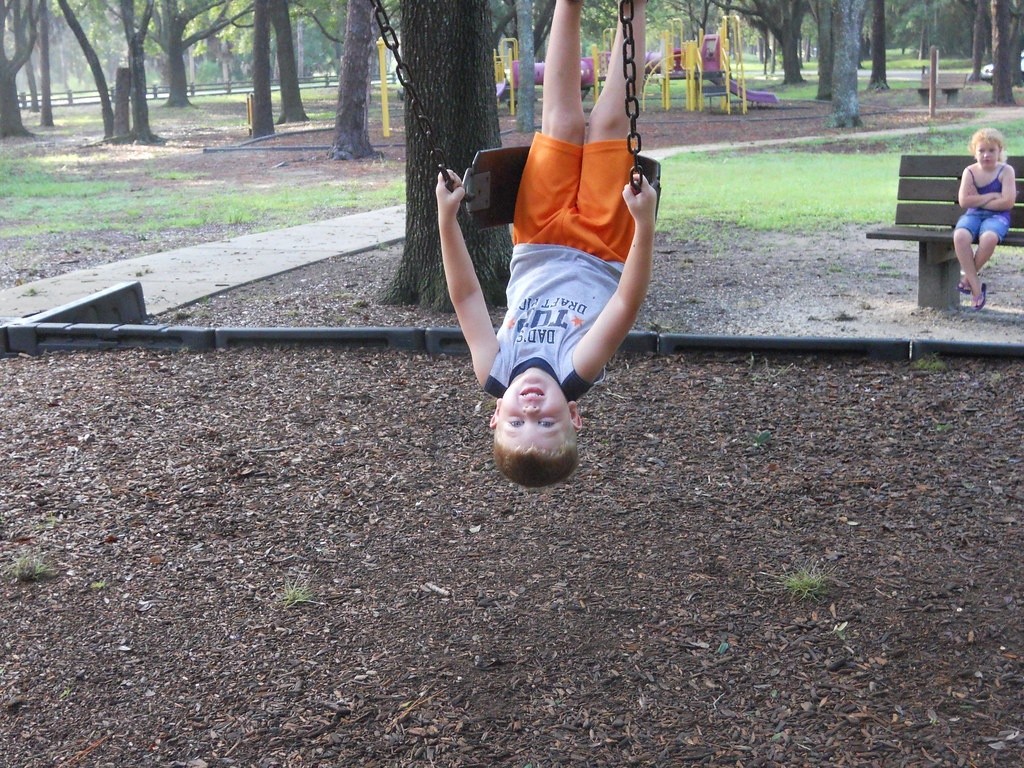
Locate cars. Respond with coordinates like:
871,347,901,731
981,51,1024,85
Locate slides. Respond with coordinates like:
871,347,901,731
496,78,507,98
707,65,778,105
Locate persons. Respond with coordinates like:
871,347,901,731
952,128,1016,311
436,0,658,489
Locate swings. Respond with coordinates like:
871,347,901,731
368,0,663,229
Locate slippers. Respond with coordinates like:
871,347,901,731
971,283,987,312
955,279,972,295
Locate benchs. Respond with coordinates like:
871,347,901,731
866,155,1024,310
